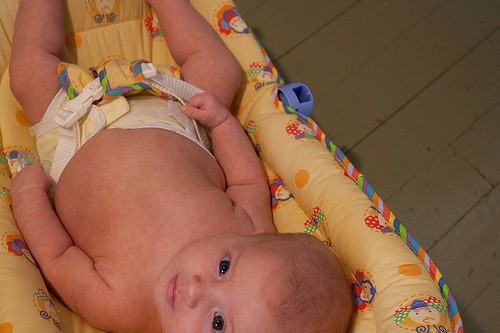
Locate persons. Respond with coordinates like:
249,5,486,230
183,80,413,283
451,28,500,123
6,0,354,333
